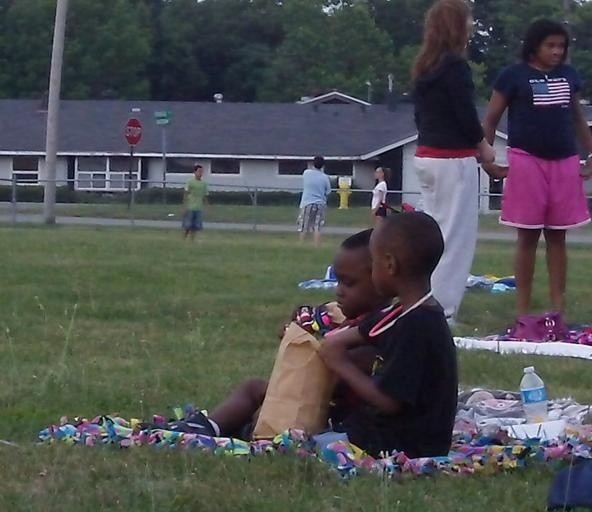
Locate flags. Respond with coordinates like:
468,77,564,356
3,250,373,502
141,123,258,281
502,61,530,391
530,82,572,107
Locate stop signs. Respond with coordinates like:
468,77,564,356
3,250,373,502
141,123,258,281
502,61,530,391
123,117,142,145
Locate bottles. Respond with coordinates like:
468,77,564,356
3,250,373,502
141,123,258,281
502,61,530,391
519,366,547,424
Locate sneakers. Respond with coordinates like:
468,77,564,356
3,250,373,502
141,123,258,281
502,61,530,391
136,412,218,437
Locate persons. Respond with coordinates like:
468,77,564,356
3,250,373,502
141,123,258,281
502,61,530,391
246,209,460,464
371,164,391,230
130,227,398,448
409,0,497,351
294,154,333,246
180,164,209,243
483,16,592,328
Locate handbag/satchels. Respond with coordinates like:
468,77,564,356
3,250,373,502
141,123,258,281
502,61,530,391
253,321,335,440
